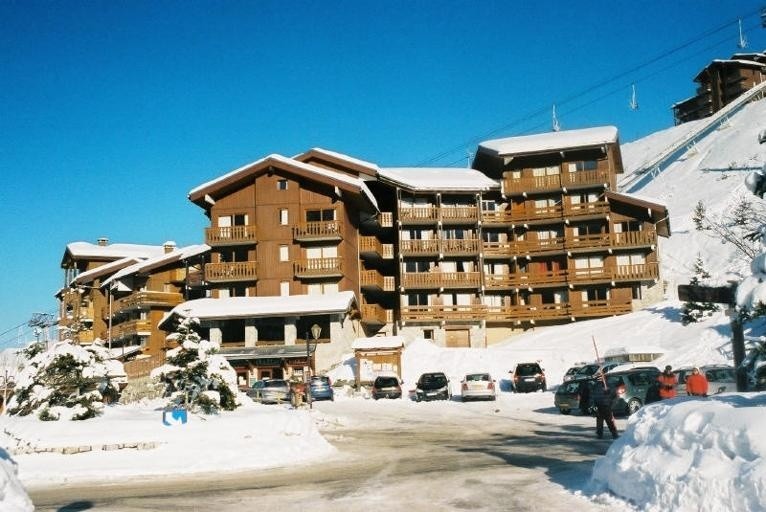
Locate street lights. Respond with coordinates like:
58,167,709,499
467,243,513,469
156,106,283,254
77,284,112,352
305,325,323,409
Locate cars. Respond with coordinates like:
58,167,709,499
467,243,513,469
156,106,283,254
371,375,401,400
555,361,662,415
461,373,496,401
509,363,546,392
737,337,766,390
414,374,453,401
304,374,333,401
247,377,293,405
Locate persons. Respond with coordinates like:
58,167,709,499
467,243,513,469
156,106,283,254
591,370,619,439
713,386,726,395
654,365,680,400
686,366,709,397
0,389,35,512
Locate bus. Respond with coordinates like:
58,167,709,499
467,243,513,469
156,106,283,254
607,348,664,364
